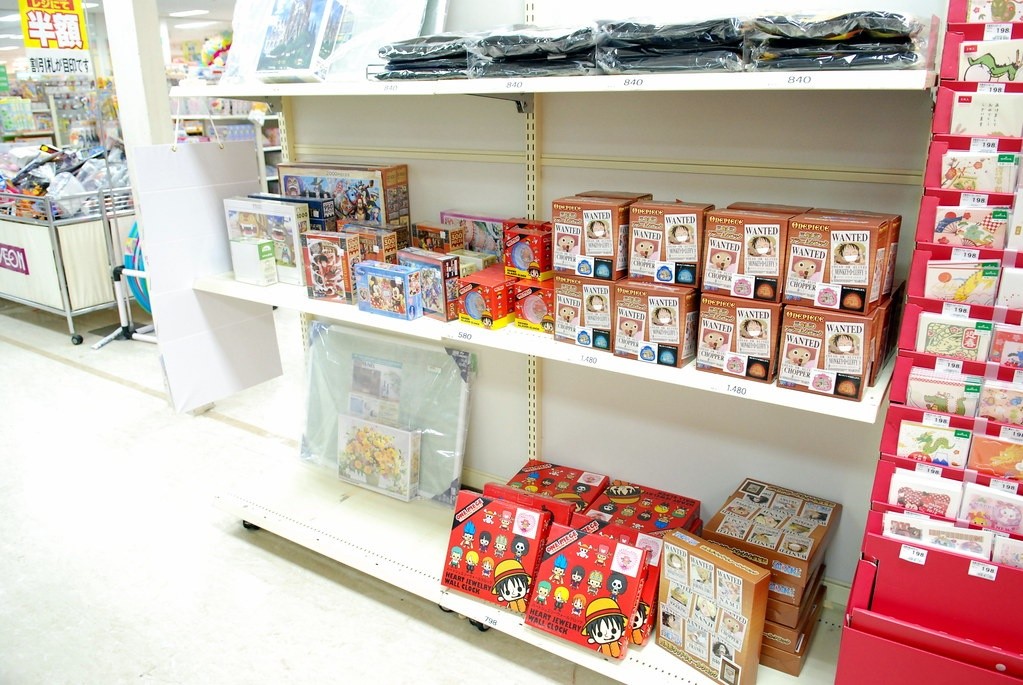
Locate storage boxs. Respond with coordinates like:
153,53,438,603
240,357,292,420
335,411,423,502
439,457,846,685
253,1,344,82
221,154,908,402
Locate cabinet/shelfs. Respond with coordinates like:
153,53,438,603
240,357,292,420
833,1,1022,685
174,52,940,684
22,94,61,147
